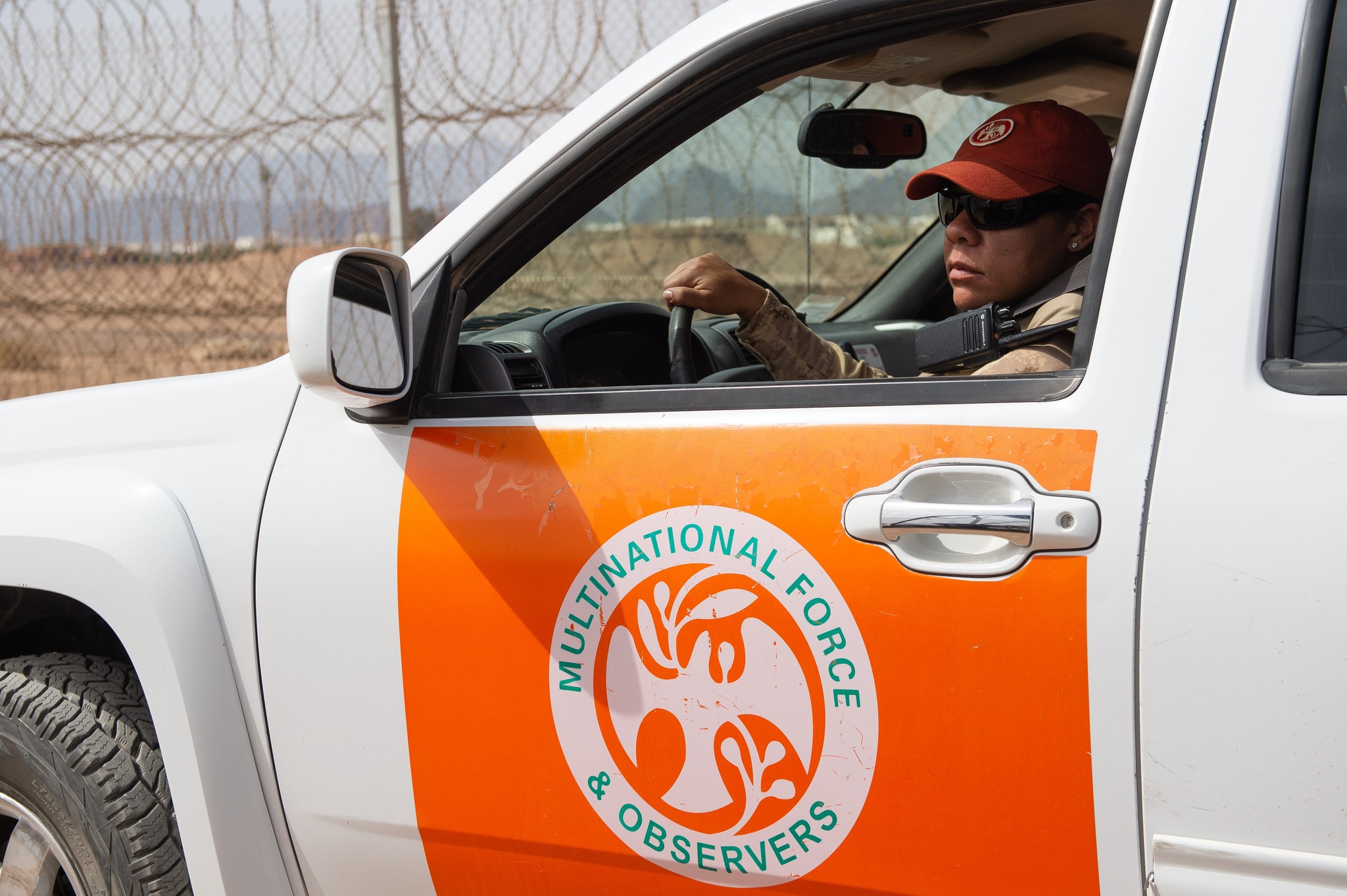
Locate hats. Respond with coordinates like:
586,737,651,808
905,99,1113,202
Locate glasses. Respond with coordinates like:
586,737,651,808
937,189,1085,231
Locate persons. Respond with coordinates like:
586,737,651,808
663,99,1112,381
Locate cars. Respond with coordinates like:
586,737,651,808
0,3,1347,896
